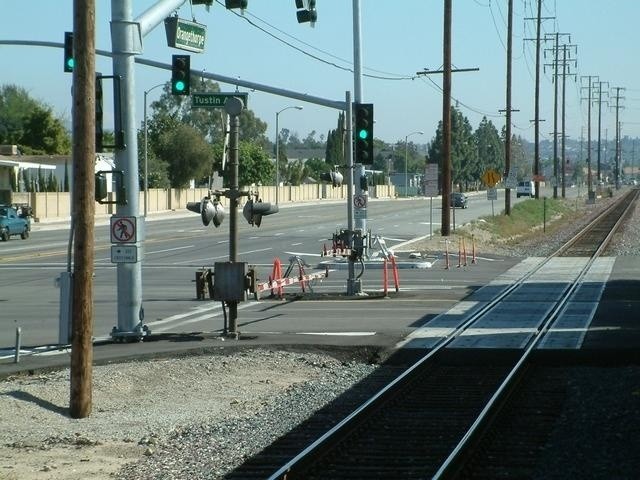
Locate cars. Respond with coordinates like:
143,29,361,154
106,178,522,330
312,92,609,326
450,190,468,210
0,206,31,242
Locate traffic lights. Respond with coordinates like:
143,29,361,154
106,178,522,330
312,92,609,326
171,54,190,96
353,102,375,166
243,198,278,227
185,195,227,227
64,31,77,73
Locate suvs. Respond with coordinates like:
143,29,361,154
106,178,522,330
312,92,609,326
515,179,537,200
